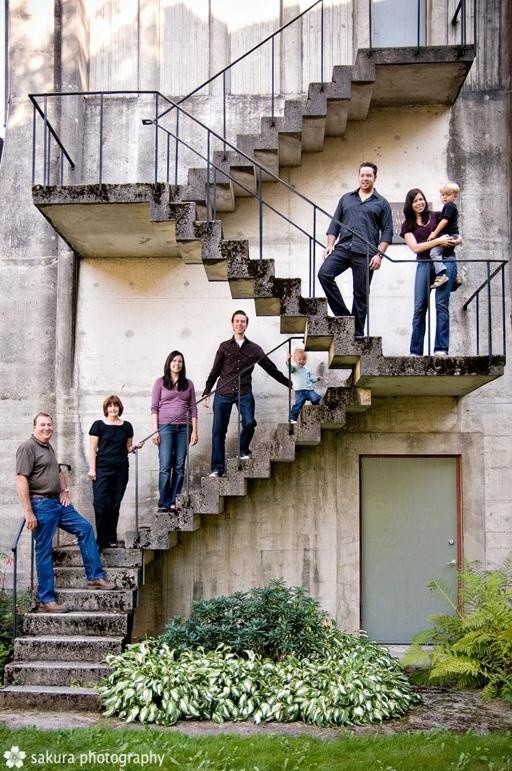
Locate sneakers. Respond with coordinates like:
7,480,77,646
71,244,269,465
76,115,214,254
97,419,298,547
435,350,446,356
431,274,449,288
451,275,462,291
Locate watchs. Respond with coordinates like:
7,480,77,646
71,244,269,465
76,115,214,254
61,487,71,493
376,252,385,260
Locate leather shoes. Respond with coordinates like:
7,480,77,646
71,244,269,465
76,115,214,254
86,579,116,590
39,600,68,613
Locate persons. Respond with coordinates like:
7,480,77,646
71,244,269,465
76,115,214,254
399,188,458,357
16,413,117,612
202,309,292,477
87,396,144,549
286,349,322,424
317,161,393,338
427,181,460,287
150,349,198,513
